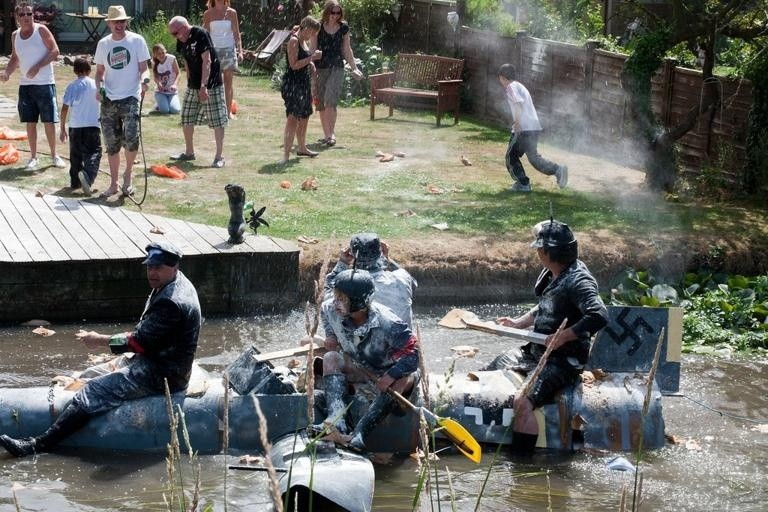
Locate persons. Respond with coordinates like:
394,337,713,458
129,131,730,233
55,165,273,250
150,44,182,114
309,1,364,147
0,3,67,173
275,16,323,171
478,200,609,457
1,242,202,459
94,5,152,198
497,64,568,193
204,1,244,121
59,57,102,196
322,233,417,332
168,15,228,170
306,250,421,449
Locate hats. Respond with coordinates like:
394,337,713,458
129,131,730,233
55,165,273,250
350,232,389,272
142,242,184,266
530,219,577,250
104,5,131,21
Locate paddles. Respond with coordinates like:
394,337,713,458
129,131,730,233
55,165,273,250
336,344,481,464
437,308,549,346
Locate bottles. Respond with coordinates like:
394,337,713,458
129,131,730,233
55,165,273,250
230,100,237,115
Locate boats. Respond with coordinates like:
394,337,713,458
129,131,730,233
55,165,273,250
0,306,683,512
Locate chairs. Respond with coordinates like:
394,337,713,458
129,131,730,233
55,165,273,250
236,27,293,77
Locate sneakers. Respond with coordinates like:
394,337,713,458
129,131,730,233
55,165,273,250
25,156,40,169
52,154,67,168
211,156,225,168
296,146,319,157
170,152,195,161
509,181,530,192
554,164,567,189
78,170,93,196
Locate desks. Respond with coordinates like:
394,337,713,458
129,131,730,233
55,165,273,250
64,13,132,56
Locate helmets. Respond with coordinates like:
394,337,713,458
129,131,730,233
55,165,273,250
324,269,385,317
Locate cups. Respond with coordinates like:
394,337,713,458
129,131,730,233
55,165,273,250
381,60,388,73
87,5,92,15
92,6,98,16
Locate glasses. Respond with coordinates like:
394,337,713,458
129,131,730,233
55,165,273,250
18,12,33,17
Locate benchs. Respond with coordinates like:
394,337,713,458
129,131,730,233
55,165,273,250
368,52,465,127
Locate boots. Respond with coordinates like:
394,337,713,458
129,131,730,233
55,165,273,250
306,372,400,453
0,401,89,458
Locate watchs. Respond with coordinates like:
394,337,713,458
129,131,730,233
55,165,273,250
200,84,208,87
